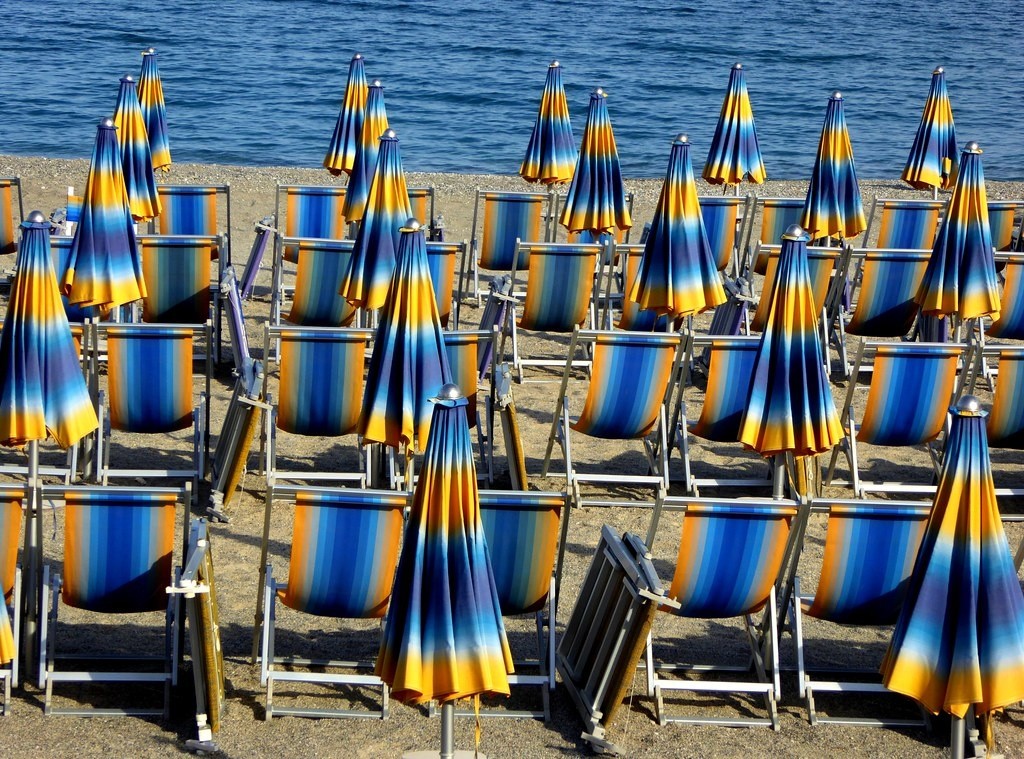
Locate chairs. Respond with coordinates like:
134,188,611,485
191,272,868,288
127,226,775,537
0,175,1024,730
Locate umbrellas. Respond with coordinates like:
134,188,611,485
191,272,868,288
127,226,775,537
558,88,632,297
324,53,371,178
519,60,579,218
60,119,148,408
340,78,391,229
878,394,1024,759
802,92,867,247
375,382,514,759
626,134,729,404
738,225,847,503
339,129,422,324
357,217,462,493
911,140,1002,393
132,46,174,177
702,63,766,220
901,65,960,201
0,209,101,678
107,74,163,232
